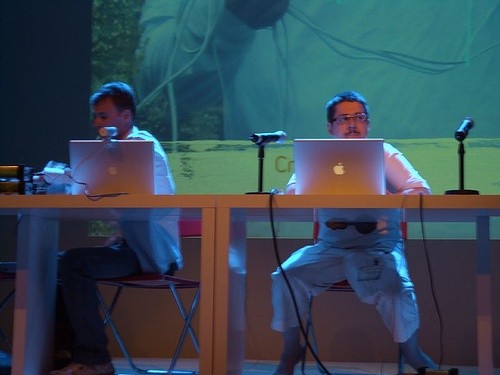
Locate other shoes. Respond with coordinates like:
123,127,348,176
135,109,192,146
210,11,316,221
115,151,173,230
273,345,304,375
405,352,437,372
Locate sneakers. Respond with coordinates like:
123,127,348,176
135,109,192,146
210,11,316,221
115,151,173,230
49,353,115,375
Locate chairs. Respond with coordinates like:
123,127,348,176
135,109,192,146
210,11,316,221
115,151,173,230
0,262,64,358
93,217,203,375
302,222,408,375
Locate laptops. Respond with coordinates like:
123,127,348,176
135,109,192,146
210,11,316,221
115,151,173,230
293,137,386,195
69,139,155,195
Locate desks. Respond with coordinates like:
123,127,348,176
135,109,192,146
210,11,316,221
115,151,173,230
0,193,500,375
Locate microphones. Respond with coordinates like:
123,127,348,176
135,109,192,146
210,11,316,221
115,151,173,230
248,130,288,144
455,117,474,141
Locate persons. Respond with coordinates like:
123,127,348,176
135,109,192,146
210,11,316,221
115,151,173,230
272,92,434,375
51,82,182,375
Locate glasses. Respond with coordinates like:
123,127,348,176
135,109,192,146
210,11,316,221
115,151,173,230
330,113,368,123
